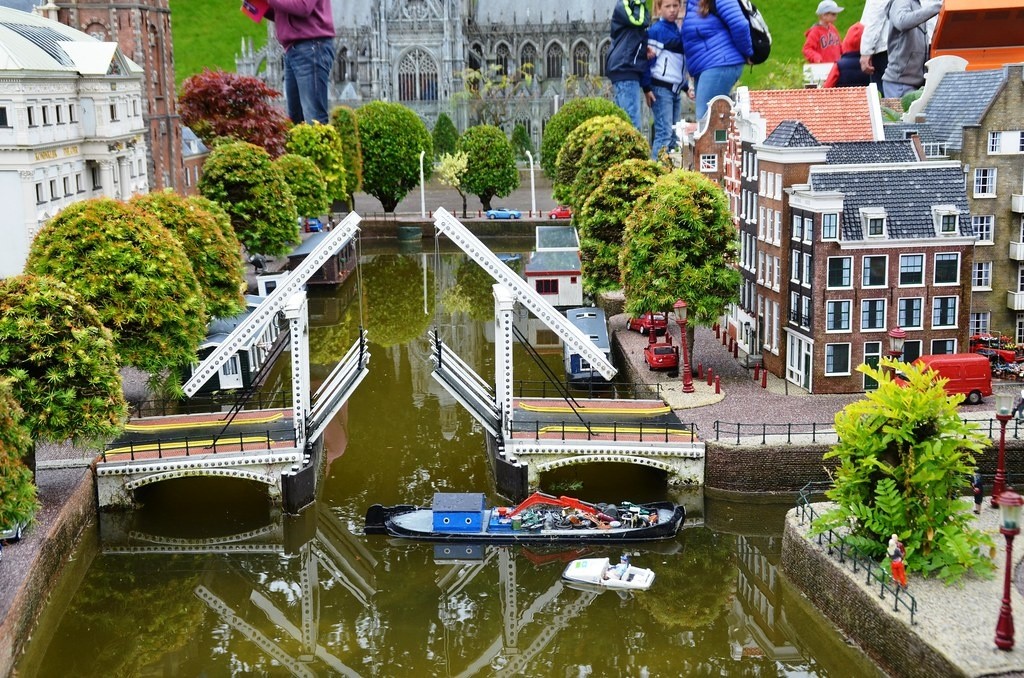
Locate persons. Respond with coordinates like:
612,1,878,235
606,0,655,134
640,0,695,171
680,0,752,127
822,0,942,100
238,0,336,127
802,0,845,90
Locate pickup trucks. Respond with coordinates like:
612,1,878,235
969,333,1024,367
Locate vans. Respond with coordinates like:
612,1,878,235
890,356,994,406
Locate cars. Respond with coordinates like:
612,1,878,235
548,204,572,220
644,343,677,371
626,312,668,336
303,219,322,231
485,207,522,220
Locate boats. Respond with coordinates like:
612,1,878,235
563,307,613,393
365,492,688,541
526,225,584,316
187,270,288,399
287,231,357,288
561,551,656,592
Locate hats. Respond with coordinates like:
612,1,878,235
816,0,844,15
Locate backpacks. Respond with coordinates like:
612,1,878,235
738,0,772,64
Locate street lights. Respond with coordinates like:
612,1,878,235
989,484,1024,651
671,298,695,393
991,392,1014,509
887,326,905,381
